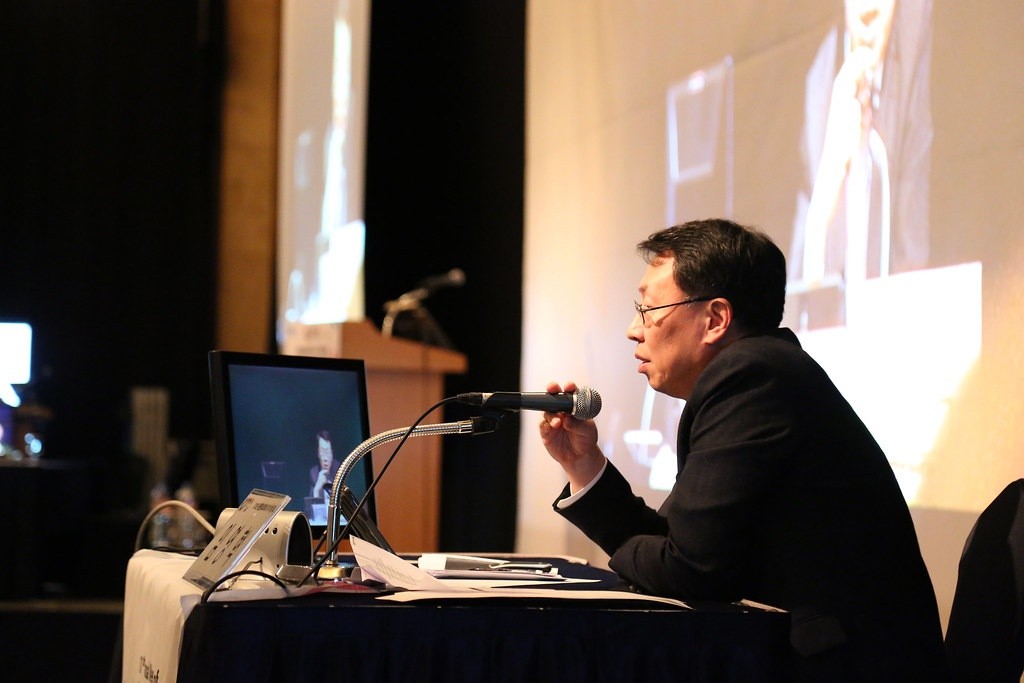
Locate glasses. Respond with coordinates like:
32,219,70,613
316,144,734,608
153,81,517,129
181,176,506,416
633,296,736,325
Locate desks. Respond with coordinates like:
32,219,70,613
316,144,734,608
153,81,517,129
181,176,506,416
130,552,792,683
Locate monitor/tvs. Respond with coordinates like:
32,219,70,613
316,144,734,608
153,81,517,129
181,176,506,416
208,350,379,538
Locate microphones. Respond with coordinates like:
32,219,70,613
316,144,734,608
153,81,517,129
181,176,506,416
401,269,466,303
456,386,603,420
855,78,874,132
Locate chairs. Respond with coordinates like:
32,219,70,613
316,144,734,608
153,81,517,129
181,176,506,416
942,477,1024,683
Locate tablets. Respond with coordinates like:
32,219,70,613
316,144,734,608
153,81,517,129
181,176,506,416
323,482,396,558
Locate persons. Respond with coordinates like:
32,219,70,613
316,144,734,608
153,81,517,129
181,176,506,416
541,219,949,683
792,0,932,280
310,429,346,522
